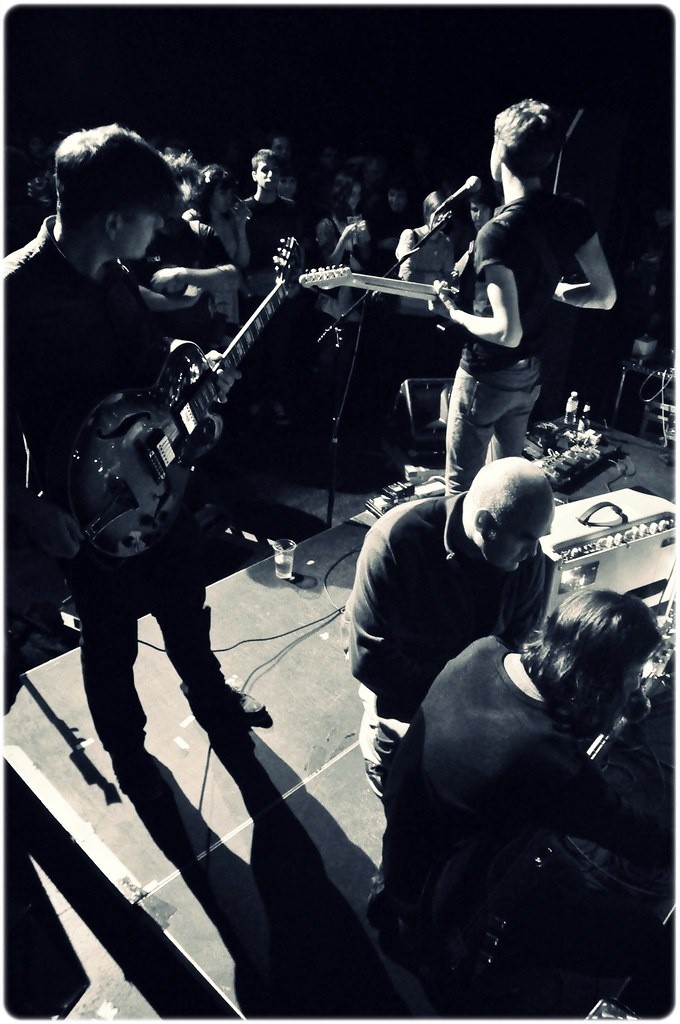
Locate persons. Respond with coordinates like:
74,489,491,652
6,99,674,791
354,456,551,721
384,592,673,870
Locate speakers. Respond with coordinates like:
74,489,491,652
391,377,454,449
538,488,676,632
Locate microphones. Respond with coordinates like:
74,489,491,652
433,175,481,216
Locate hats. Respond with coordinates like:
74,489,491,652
196,163,240,189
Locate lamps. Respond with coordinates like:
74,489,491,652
391,377,456,462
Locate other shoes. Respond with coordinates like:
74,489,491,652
180,684,267,717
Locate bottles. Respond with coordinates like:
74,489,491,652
564,391,579,425
577,401,591,433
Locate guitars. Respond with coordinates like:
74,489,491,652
583,613,675,766
54,233,308,560
296,262,460,306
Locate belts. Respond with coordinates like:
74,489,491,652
504,355,544,372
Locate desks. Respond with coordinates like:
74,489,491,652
611,351,675,428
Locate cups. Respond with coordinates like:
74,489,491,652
272,539,296,579
347,215,362,225
230,196,252,225
